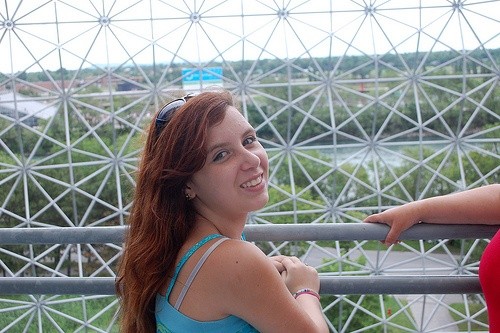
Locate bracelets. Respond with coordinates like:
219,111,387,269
291,288,321,300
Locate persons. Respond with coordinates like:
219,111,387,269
119,89,331,333
361,183,500,333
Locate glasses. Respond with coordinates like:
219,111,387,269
154,92,202,142
